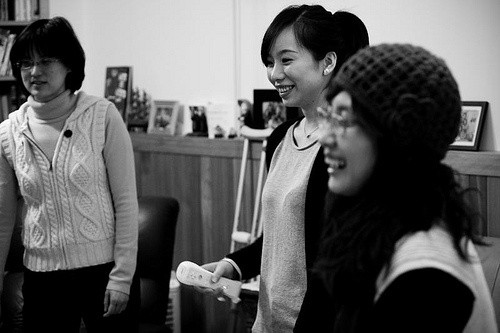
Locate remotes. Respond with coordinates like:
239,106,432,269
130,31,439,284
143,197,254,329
176,260,242,300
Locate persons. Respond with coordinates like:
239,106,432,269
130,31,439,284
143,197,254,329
309,43,498,333
1,17,139,333
193,5,370,333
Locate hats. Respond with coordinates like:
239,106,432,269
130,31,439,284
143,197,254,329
333,43,461,159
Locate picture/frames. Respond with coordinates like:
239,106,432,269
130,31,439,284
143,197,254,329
103,66,489,151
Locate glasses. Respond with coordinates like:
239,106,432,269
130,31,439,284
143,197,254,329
16,58,59,70
314,106,367,140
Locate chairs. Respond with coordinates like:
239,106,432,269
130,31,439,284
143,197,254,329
0,195,500,333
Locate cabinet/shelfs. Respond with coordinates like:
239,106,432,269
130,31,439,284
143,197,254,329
0,0,50,124
